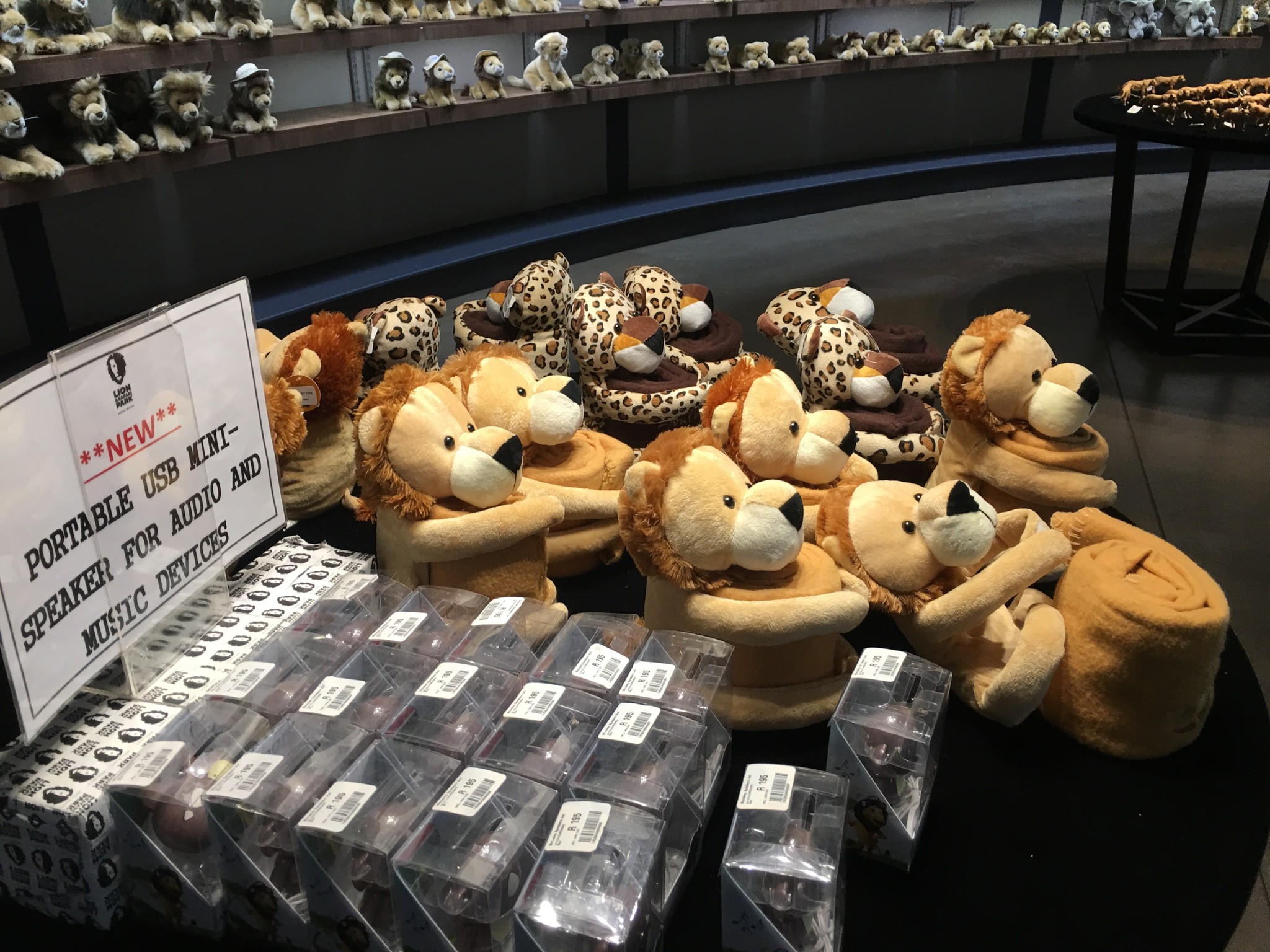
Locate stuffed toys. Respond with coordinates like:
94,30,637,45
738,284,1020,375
813,473,1073,727
923,307,1119,532
757,274,948,469
564,271,760,425
617,424,871,729
0,0,277,180
349,295,446,418
437,340,634,577
256,309,370,519
354,362,568,645
286,0,1270,137
699,355,879,543
621,265,744,362
452,252,606,432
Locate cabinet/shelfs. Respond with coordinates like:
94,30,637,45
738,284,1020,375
0,0,1270,393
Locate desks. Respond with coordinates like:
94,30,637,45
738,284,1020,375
0,480,1270,952
1074,91,1270,357
